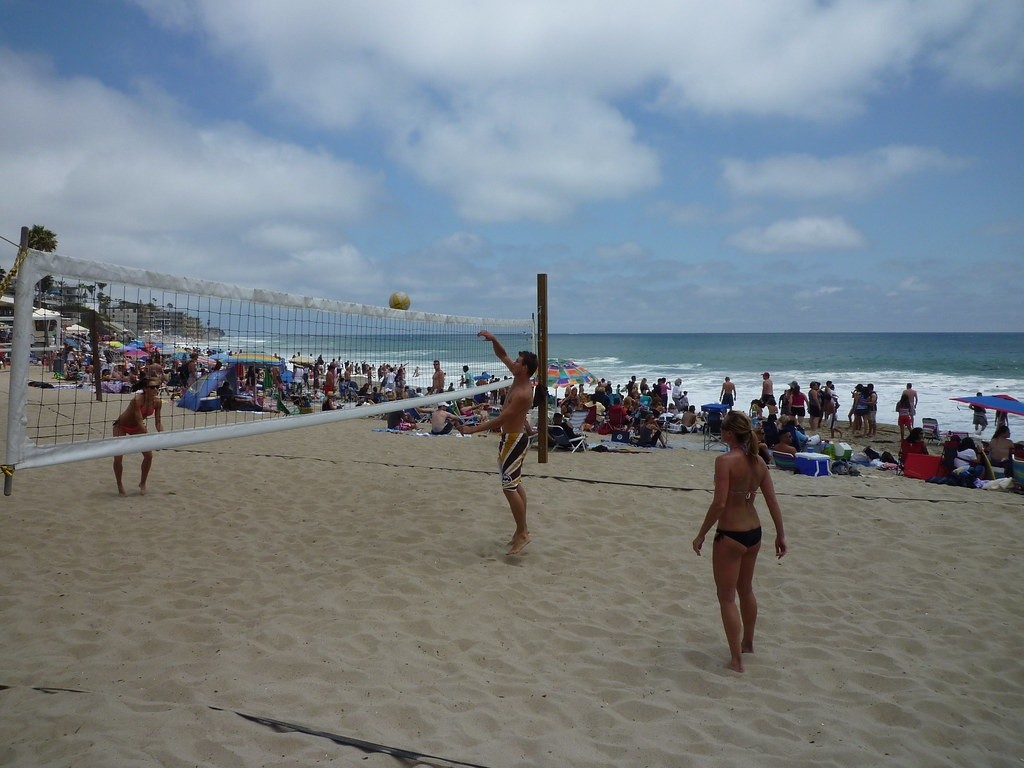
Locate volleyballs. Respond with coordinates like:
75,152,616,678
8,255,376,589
389,291,411,311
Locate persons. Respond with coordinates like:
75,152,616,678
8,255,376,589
478,332,539,555
692,410,788,674
54,332,1024,496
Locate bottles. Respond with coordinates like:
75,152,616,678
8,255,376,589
819,439,836,459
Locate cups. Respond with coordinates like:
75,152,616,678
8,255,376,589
807,447,814,453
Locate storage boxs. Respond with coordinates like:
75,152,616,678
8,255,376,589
835,441,853,459
200,396,222,414
795,452,832,476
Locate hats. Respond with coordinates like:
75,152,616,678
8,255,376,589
788,380,797,388
385,388,392,392
438,402,450,407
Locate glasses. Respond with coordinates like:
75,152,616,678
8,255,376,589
147,385,159,390
433,363,438,366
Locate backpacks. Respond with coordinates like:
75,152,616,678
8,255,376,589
181,361,195,380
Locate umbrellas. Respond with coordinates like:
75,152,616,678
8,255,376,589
948,394,1024,434
530,357,599,416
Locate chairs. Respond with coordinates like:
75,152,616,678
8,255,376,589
274,394,1024,491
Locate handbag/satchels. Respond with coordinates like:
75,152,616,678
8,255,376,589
969,462,977,467
612,424,630,442
597,422,612,436
